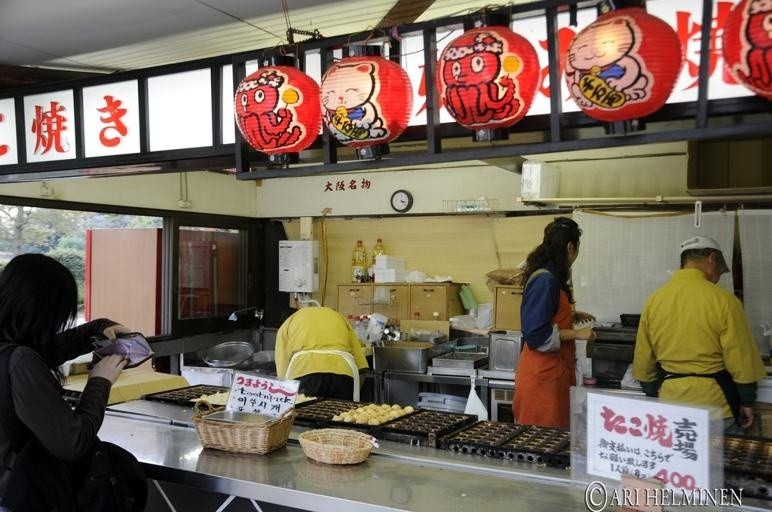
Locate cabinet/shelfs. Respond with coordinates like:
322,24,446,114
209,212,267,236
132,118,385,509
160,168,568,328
494,285,523,331
335,282,469,322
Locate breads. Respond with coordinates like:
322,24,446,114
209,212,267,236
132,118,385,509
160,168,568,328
333,399,416,427
195,382,317,409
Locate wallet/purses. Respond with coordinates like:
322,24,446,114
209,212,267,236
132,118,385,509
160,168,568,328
87,331,155,370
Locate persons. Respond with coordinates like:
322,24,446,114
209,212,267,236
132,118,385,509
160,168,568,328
632,237,768,435
1,253,135,511
513,216,597,427
275,301,370,403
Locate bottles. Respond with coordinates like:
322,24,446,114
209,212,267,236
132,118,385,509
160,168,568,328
353,314,361,339
361,316,369,339
371,238,386,267
346,315,353,327
350,241,368,284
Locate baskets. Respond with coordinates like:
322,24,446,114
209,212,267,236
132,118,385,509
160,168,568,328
192,398,297,455
298,428,378,466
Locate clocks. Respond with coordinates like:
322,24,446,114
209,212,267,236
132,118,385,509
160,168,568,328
391,190,413,213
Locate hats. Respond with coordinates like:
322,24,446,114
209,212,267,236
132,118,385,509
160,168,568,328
680,235,730,273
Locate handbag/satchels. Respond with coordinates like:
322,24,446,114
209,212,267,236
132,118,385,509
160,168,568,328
1,434,149,512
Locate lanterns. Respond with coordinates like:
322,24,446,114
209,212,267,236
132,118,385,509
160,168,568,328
321,55,412,159
723,0,771,98
563,9,681,133
232,66,322,164
434,24,540,141
